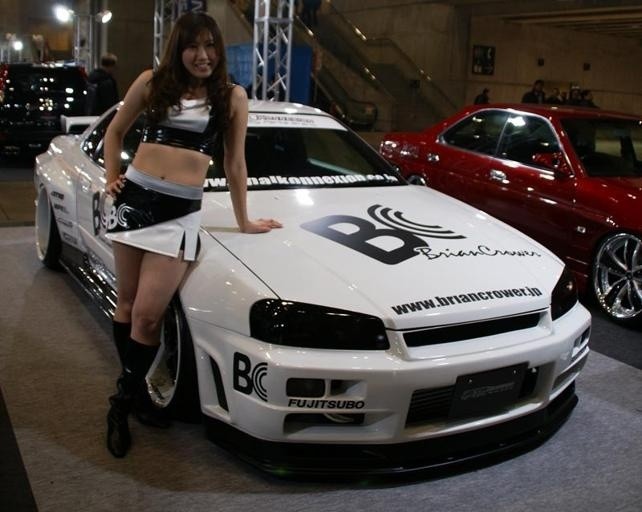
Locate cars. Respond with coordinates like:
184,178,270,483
380,100,641,329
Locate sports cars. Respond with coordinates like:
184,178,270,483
31,95,593,478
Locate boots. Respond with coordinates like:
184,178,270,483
104,336,162,459
111,317,173,429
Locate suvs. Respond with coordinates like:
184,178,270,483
0,60,97,166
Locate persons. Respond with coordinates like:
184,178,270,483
518,77,600,110
473,86,491,105
104,12,285,458
85,52,121,117
